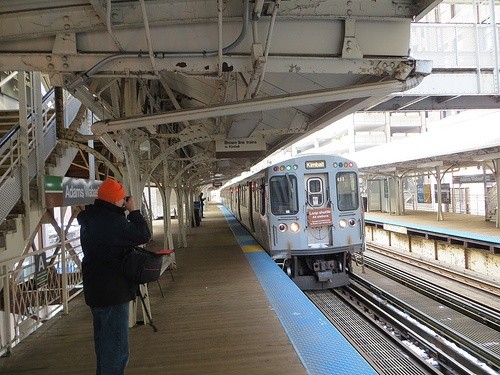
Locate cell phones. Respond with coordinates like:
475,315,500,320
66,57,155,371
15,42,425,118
123,197,129,206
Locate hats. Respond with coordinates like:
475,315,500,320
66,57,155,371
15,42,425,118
97,178,126,203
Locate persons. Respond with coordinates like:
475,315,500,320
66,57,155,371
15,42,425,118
199,193,206,218
77,177,152,375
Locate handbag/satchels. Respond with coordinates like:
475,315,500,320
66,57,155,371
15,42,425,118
120,244,161,283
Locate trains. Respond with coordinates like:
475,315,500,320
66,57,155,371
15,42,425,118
220,153,365,291
